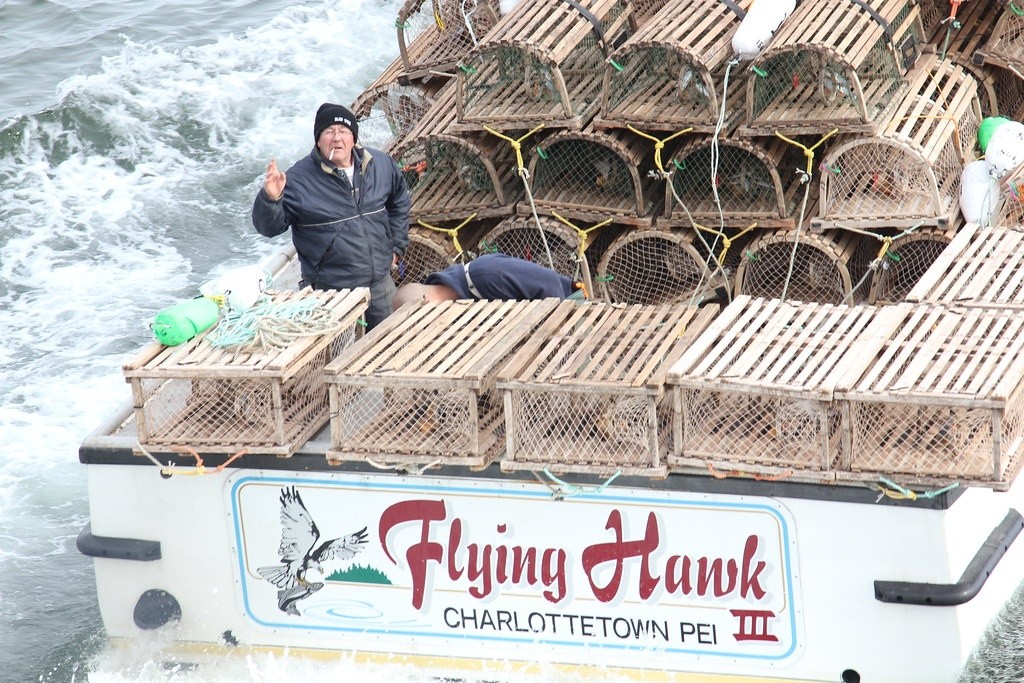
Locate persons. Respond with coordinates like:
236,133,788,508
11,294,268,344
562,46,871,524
390,254,593,338
251,104,412,333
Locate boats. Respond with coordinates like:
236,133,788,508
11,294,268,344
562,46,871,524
73,0,1023,683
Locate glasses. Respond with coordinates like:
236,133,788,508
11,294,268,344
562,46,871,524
322,128,353,136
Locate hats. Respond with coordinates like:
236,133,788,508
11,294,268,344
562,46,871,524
314,103,358,144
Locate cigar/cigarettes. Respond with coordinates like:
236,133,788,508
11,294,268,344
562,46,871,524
329,147,335,160
